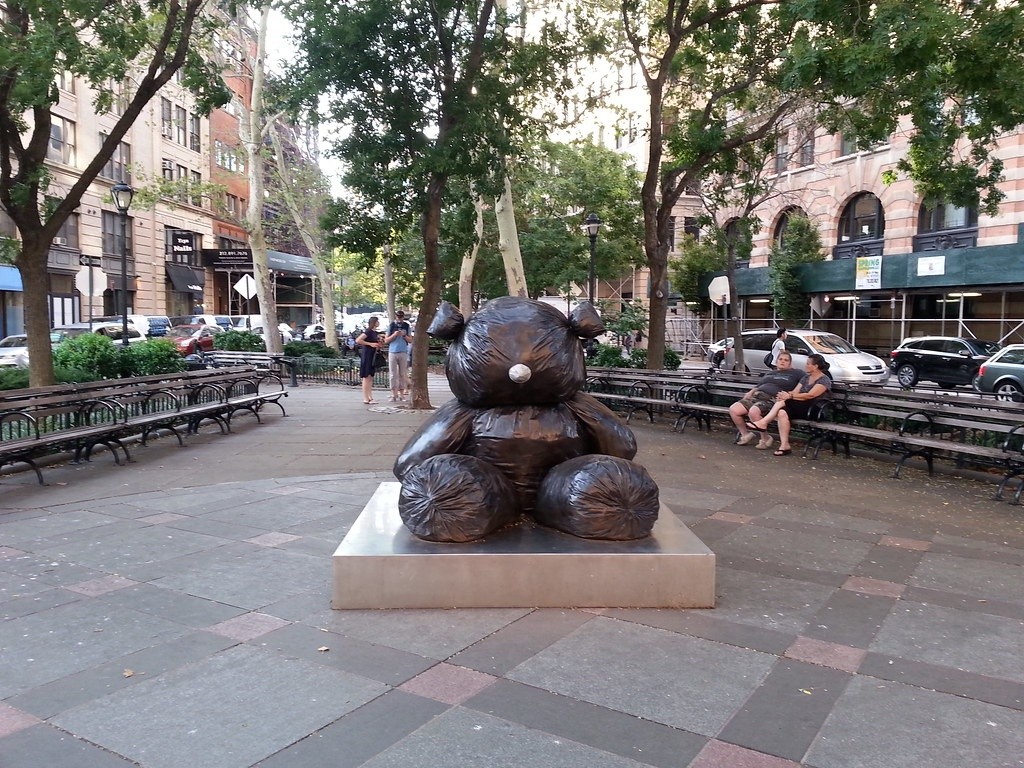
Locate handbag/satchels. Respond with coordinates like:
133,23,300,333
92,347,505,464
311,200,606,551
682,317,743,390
764,353,775,370
372,348,386,368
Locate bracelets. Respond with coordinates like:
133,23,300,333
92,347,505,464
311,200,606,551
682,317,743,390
791,393,794,399
402,334,407,337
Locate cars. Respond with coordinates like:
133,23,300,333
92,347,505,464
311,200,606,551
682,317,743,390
49,322,147,345
707,327,891,387
88,314,303,345
160,324,222,357
0,334,79,372
890,336,1024,403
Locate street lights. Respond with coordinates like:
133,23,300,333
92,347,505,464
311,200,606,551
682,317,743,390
581,210,603,360
110,181,139,344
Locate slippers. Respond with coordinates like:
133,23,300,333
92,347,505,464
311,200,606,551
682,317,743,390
773,450,792,456
746,421,766,432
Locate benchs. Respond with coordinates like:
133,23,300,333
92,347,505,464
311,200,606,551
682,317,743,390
0,364,289,488
201,349,285,383
583,367,1024,505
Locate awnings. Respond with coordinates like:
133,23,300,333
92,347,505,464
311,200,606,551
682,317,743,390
0,262,23,291
165,265,204,294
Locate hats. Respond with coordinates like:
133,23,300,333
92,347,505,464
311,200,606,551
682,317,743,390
394,311,404,315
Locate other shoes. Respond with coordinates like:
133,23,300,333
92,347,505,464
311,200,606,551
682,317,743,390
400,396,407,402
737,432,754,445
389,397,396,402
756,435,773,449
364,399,379,403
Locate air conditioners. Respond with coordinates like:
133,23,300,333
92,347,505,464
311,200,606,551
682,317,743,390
53,237,67,245
162,127,172,137
162,161,172,170
224,57,236,67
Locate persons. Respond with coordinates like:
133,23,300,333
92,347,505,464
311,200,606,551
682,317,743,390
744,354,833,457
729,352,808,448
384,311,413,402
770,328,788,370
355,317,384,404
